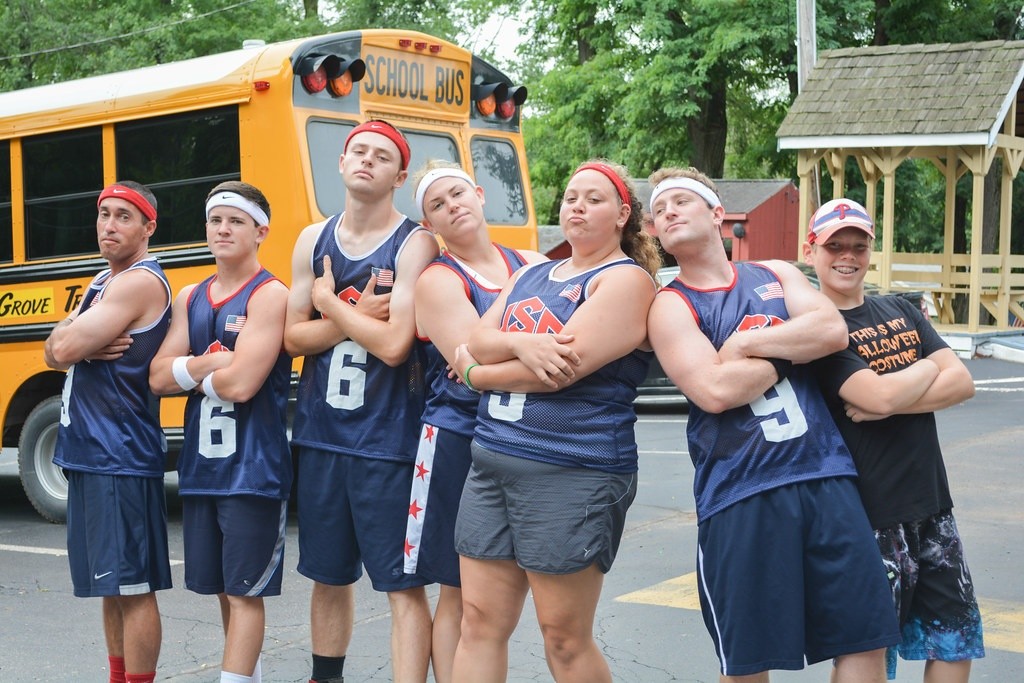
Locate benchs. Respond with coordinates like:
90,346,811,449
890,287,1024,325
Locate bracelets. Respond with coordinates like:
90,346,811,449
203,371,222,402
173,355,199,392
464,364,482,390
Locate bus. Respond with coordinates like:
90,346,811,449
0,28,541,532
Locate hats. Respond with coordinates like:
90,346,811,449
808,199,875,245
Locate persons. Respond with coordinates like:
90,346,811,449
281,119,433,683
402,155,554,683
44,179,172,683
446,161,657,683
645,164,900,683
797,197,985,682
147,180,297,683
